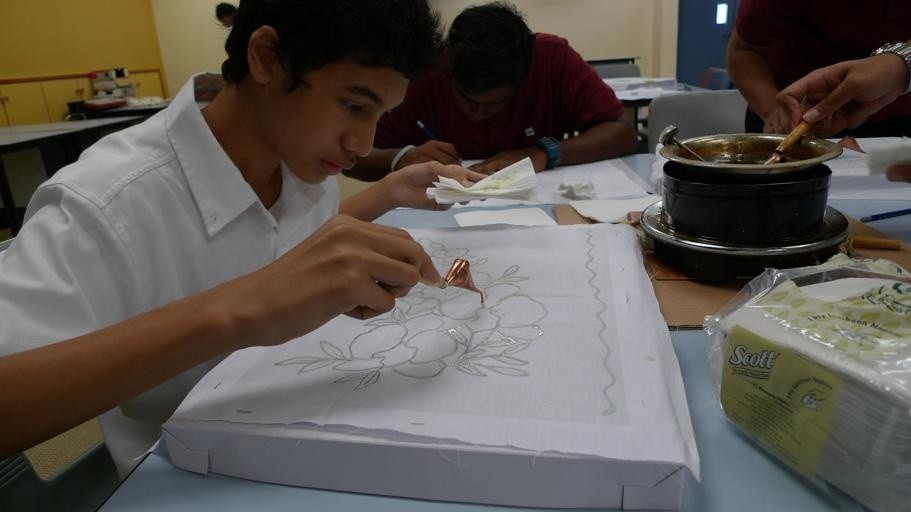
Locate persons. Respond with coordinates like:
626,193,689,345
340,1,639,179
0,0,489,483
725,0,911,137
775,38,911,147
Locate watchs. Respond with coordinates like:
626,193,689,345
536,136,561,170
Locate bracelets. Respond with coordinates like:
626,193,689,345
390,143,415,172
870,41,911,96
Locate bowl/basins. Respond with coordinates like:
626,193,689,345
661,161,832,237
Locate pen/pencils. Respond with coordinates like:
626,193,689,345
417,121,463,165
860,207,910,223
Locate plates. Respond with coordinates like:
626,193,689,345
639,197,853,271
659,132,843,182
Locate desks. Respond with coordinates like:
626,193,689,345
0,78,911,512
